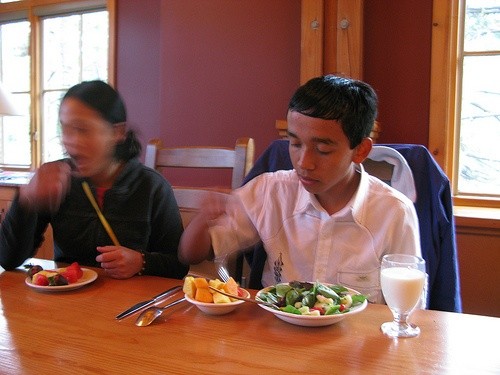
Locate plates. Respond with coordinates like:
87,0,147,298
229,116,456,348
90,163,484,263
24,267,99,292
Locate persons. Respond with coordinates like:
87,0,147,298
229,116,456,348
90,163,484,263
177,72,429,312
0,78,193,280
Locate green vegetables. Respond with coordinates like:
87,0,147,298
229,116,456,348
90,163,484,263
260,279,366,316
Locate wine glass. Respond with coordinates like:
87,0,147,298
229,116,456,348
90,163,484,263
378,252,426,340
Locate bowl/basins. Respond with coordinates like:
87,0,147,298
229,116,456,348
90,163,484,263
254,281,368,327
184,287,252,315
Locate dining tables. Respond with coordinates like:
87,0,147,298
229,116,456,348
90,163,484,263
0,245,500,375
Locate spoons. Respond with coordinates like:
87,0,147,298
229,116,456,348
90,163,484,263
135,296,186,327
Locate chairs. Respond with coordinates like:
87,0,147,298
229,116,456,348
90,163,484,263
140,136,255,269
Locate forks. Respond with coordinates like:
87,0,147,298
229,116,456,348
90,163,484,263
216,219,237,285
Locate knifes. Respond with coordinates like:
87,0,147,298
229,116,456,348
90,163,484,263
114,285,183,321
